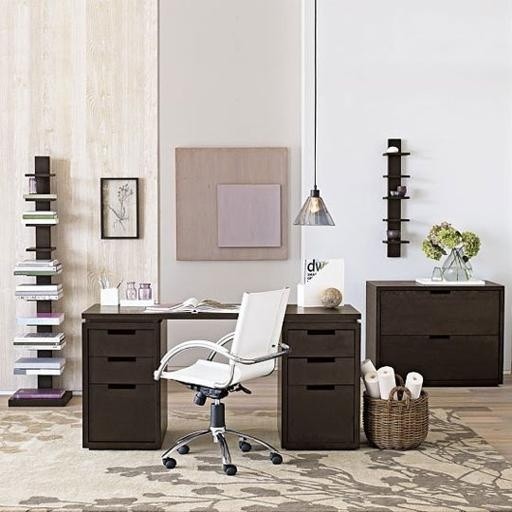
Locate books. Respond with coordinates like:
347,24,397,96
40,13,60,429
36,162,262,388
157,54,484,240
13,388,66,400
21,219,59,225
11,260,68,377
148,298,241,314
303,258,334,283
22,210,56,216
22,214,57,219
23,193,57,198
145,302,184,311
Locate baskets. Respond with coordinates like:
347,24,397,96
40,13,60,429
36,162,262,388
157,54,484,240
363,374,429,451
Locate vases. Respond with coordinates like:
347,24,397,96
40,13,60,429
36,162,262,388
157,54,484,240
443,248,473,282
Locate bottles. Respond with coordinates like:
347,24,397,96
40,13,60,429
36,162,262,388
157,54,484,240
431,266,443,282
126,281,138,300
138,282,152,301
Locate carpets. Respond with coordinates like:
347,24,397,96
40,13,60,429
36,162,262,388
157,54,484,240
0,407,512,512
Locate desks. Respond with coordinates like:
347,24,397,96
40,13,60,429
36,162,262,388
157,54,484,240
81,302,363,450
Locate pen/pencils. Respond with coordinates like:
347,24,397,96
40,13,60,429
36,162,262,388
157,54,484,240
98,277,124,289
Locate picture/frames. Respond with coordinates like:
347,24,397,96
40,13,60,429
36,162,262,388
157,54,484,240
99,177,139,240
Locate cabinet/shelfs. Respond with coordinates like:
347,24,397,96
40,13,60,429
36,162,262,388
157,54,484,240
382,138,411,257
366,280,505,388
8,155,73,407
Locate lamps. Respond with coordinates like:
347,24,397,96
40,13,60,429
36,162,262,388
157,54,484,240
292,0,336,228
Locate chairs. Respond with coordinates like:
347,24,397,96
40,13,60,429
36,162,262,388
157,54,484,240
153,285,293,475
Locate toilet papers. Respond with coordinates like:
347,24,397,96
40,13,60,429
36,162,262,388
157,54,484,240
361,359,424,400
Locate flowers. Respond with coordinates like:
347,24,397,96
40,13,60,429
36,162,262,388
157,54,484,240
423,221,481,282
